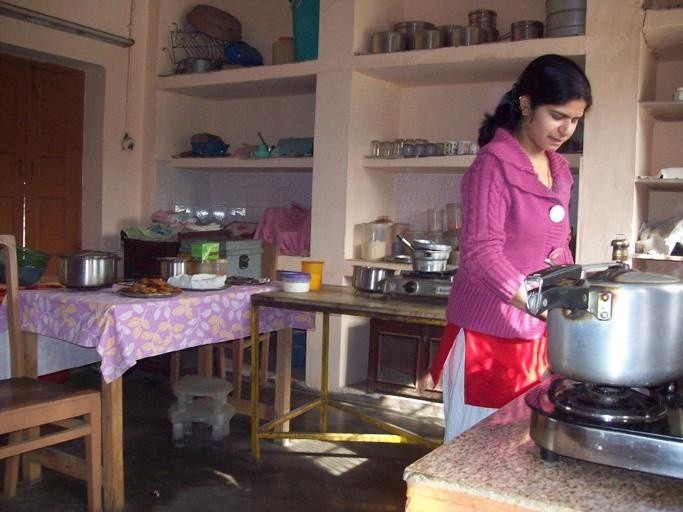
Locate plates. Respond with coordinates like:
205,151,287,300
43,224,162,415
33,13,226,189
341,0,586,278
142,1,322,265
628,0,683,280
366,319,444,403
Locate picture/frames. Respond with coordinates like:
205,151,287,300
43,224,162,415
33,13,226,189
247,284,455,461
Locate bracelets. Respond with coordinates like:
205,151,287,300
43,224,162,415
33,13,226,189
224,40,263,66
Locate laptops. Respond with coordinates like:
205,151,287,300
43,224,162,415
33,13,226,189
119,277,182,295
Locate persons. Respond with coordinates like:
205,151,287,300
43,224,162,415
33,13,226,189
430,54,592,444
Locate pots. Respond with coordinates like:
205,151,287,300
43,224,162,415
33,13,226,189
522,233,683,387
411,239,452,272
351,264,395,295
57,250,122,290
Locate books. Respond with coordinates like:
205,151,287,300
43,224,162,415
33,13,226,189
179,237,266,280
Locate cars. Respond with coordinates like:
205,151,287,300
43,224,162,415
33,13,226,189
368,138,476,159
301,261,324,292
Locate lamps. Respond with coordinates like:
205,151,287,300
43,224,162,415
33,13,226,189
0,234,102,512
168,242,279,397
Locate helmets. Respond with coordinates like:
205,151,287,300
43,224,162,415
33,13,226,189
116,289,182,298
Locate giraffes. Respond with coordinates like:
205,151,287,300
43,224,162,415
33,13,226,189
382,272,455,298
524,373,682,480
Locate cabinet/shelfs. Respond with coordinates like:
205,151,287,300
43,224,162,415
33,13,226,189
404,203,461,250
